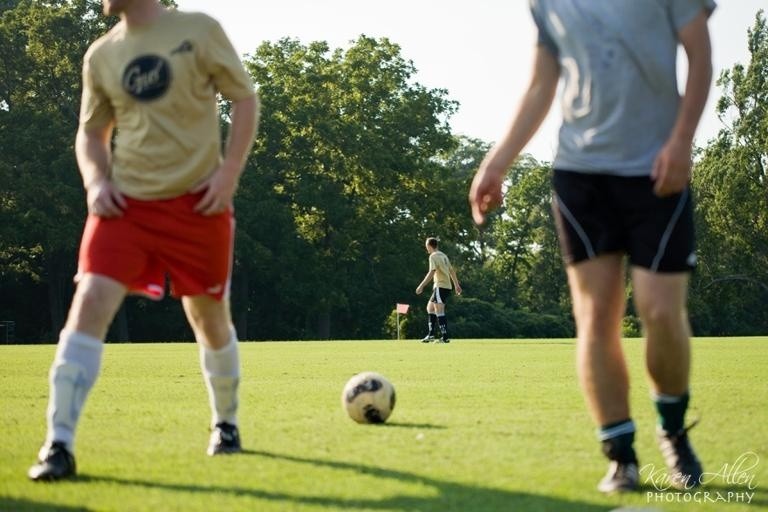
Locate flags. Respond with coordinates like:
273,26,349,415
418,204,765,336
396,303,409,315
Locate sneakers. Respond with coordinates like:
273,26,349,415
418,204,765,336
207,422,240,457
30,444,75,480
421,335,434,342
437,338,449,344
601,461,641,492
658,425,702,487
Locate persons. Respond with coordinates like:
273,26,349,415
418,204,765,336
414,237,462,345
25,0,260,486
462,0,718,496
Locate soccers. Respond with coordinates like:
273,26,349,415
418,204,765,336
344,373,395,424
